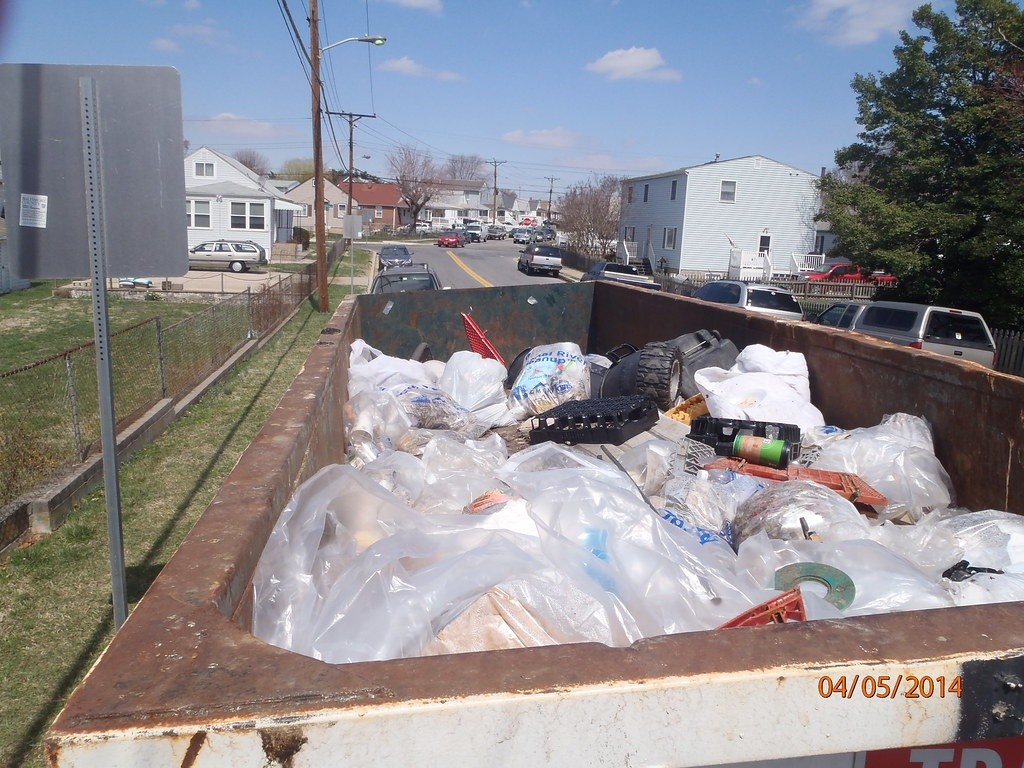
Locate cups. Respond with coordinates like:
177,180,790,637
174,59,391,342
732,434,791,471
349,411,373,447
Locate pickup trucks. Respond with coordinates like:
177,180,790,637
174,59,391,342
581,262,662,293
808,299,999,373
791,262,899,292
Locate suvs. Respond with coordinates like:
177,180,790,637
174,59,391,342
397,222,432,236
689,280,804,322
518,242,563,278
187,239,268,273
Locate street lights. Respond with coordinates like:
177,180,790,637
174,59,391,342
346,154,371,245
307,35,388,314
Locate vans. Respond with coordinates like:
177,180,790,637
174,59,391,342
467,223,489,242
487,223,507,240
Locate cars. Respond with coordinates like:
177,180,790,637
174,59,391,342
457,230,472,243
370,261,443,291
533,230,548,242
437,229,466,247
509,228,520,238
542,229,557,242
376,243,415,270
512,228,537,245
